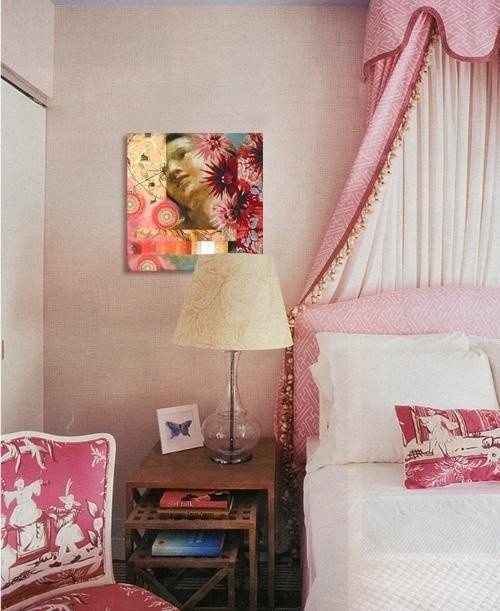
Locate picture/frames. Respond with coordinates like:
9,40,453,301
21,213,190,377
156,404,205,455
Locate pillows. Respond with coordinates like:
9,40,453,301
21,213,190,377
311,332,494,465
395,404,500,489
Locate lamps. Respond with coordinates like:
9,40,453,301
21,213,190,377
174,253,293,465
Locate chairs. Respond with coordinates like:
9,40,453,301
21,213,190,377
1,431,178,610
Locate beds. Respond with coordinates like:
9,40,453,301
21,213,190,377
298,284,497,611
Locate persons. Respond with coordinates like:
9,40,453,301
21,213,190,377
417,408,460,457
1,478,85,590
166,133,216,230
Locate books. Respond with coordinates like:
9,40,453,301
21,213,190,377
151,531,226,556
157,491,234,519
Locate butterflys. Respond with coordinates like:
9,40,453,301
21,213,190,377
165,419,193,440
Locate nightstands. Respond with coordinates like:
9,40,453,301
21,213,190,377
127,438,277,608
